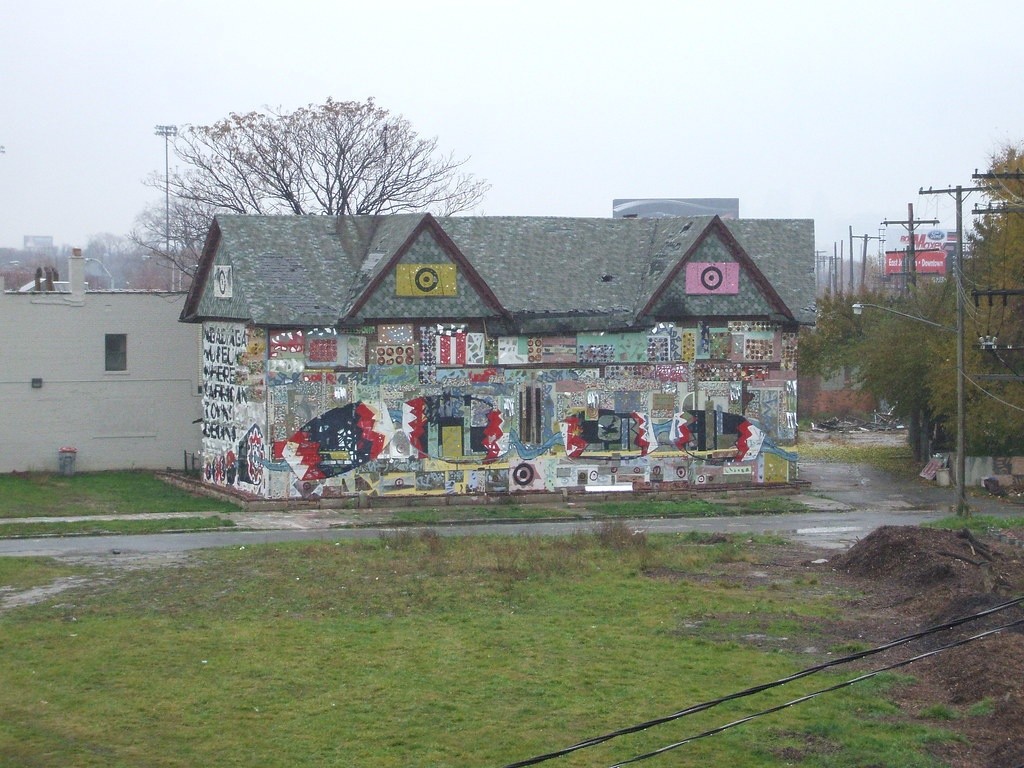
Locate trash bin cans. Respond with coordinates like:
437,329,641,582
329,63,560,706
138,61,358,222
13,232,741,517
936,468,950,486
58,447,78,477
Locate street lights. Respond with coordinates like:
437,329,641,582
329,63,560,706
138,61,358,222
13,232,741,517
155,124,178,294
86,258,115,289
851,302,966,518
179,265,199,290
